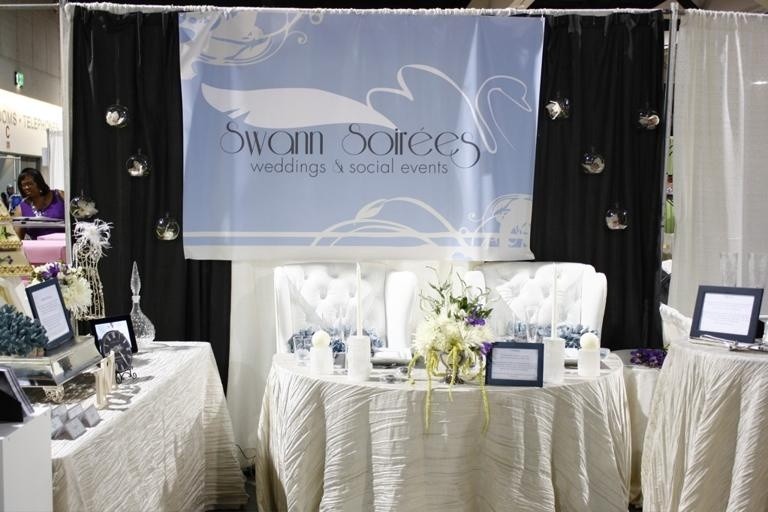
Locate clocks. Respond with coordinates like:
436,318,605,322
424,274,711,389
100,330,138,382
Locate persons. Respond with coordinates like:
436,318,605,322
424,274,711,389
7,184,23,215
14,167,65,241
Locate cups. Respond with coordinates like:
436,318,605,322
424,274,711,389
524,304,540,342
347,335,371,382
311,348,334,376
292,335,313,367
543,339,565,383
719,251,739,288
746,252,767,288
577,348,601,378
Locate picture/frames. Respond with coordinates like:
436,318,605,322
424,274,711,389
25,279,72,349
485,342,545,389
689,285,764,343
90,315,140,355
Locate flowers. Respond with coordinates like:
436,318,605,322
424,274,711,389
32,260,95,319
404,262,501,434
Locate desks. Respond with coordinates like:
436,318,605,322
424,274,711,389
50,339,252,512
250,344,633,511
636,331,768,512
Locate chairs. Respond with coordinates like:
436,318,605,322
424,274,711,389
386,266,488,356
510,259,608,347
272,260,387,355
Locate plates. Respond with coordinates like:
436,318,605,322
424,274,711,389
369,356,413,365
564,348,579,367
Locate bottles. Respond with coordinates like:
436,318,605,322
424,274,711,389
129,261,155,354
